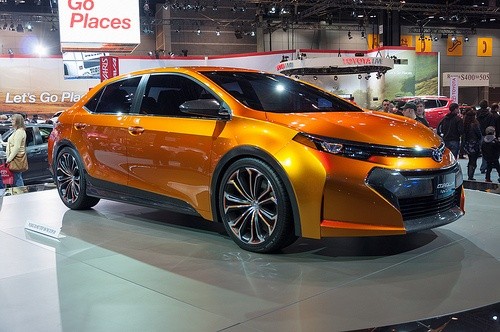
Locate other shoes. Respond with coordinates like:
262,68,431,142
485,178,500,182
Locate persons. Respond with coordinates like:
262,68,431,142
350,96,500,182
1,112,54,124
6,114,27,187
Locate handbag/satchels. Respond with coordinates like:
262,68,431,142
8,153,28,171
0,163,14,184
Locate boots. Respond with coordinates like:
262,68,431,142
467,167,476,180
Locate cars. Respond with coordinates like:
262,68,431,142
47,63,467,255
373,91,453,135
0,119,59,190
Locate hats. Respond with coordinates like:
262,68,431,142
484,126,496,142
33,114,38,119
400,102,417,111
